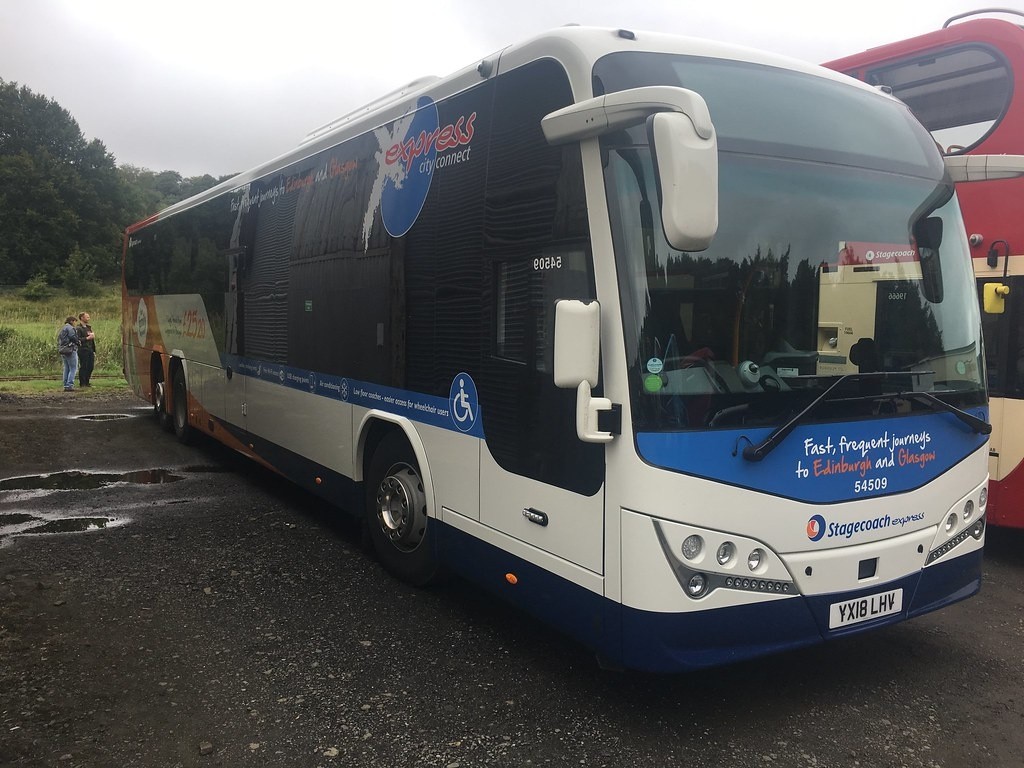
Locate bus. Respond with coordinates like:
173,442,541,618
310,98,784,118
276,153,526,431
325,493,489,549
816,8,1024,528
120,24,1024,668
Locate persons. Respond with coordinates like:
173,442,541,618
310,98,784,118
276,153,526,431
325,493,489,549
75,312,96,387
56,316,81,391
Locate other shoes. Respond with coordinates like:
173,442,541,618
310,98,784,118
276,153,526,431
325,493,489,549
80,383,91,387
65,387,77,392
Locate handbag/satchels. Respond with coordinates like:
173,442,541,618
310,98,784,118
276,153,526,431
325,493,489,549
58,343,74,354
